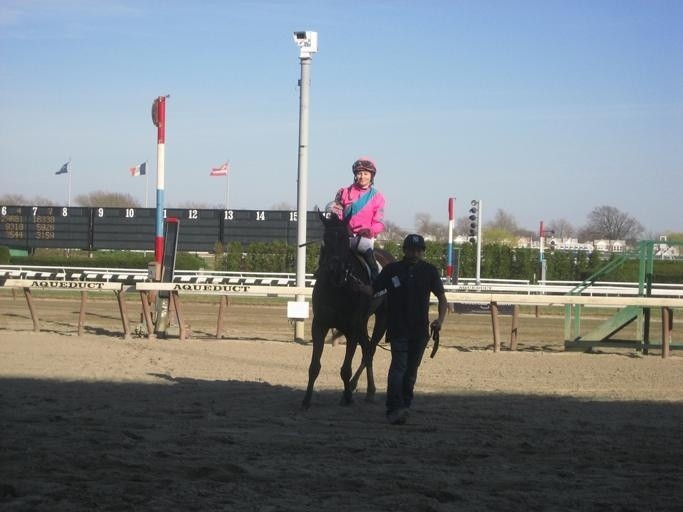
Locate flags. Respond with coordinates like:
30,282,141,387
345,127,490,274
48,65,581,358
128,161,146,177
210,160,228,176
54,160,70,174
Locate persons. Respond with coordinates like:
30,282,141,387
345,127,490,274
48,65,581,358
325,157,386,283
374,234,448,426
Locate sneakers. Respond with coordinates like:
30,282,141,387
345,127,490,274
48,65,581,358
389,408,405,424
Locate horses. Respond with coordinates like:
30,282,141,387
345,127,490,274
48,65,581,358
300,206,399,411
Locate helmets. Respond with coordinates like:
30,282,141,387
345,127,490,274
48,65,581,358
352,158,376,172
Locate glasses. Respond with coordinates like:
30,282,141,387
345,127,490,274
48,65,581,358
352,161,375,170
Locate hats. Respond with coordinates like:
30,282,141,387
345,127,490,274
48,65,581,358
404,234,426,251
